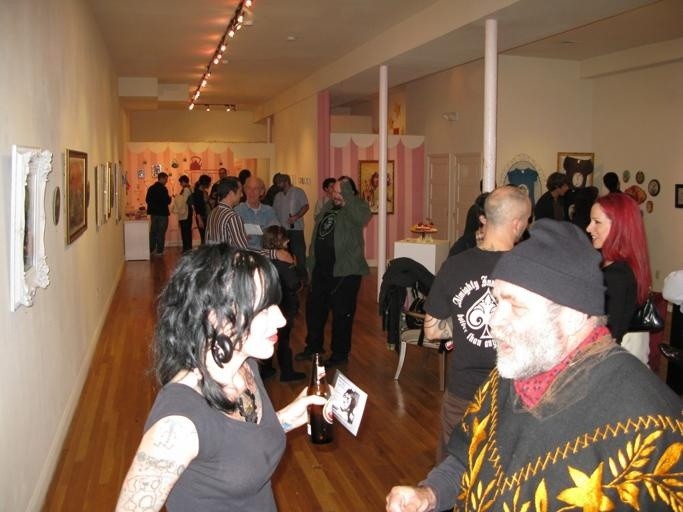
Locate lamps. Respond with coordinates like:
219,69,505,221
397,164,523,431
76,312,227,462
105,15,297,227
189,0,253,112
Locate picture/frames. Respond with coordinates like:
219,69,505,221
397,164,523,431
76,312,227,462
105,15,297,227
648,179,660,196
500,153,547,232
556,152,595,186
675,184,683,208
97,160,121,227
65,148,89,245
11,144,52,312
357,159,396,214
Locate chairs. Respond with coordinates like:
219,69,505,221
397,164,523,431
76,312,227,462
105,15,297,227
385,258,449,391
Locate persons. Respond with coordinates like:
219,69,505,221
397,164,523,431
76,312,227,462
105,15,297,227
239,169,251,202
262,172,283,207
233,177,281,250
273,174,311,293
314,178,336,221
146,172,172,256
294,176,373,365
172,175,193,251
575,186,599,231
603,172,622,194
586,193,653,372
210,168,227,207
534,172,569,219
262,225,307,382
424,185,532,451
205,176,296,264
340,389,360,424
190,174,211,244
115,242,334,512
385,218,683,512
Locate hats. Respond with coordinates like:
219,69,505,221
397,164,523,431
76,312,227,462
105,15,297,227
491,217,605,317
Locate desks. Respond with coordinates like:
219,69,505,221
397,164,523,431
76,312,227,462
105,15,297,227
123,220,151,260
394,238,450,276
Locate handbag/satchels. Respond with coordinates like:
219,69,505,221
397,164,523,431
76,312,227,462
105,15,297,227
628,288,664,331
404,286,425,328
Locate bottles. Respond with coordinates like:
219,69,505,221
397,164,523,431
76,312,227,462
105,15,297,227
289,214,294,228
307,353,334,445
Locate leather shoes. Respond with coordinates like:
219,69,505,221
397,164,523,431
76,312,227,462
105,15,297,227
280,372,306,381
295,350,313,362
